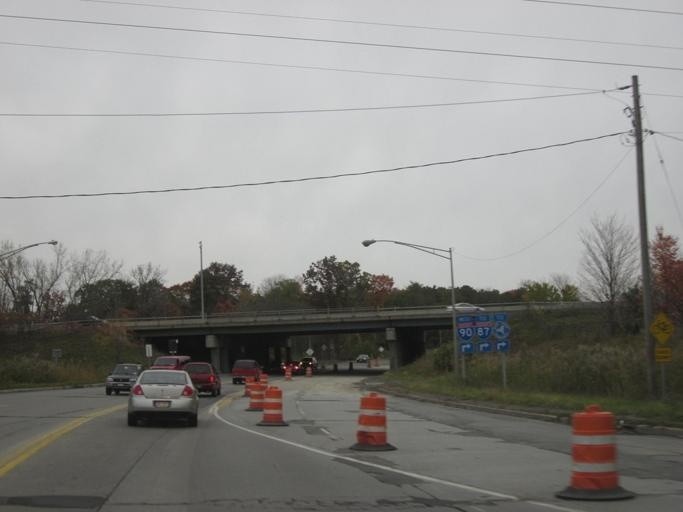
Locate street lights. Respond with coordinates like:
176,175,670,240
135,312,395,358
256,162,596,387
198,240,204,321
360,238,459,377
0,239,57,263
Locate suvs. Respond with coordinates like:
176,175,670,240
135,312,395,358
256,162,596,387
232,359,263,384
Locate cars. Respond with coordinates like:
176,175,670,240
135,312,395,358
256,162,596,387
280,357,317,375
356,353,370,364
104,355,221,428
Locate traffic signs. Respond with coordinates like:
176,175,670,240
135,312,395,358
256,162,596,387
456,315,476,342
476,314,492,339
